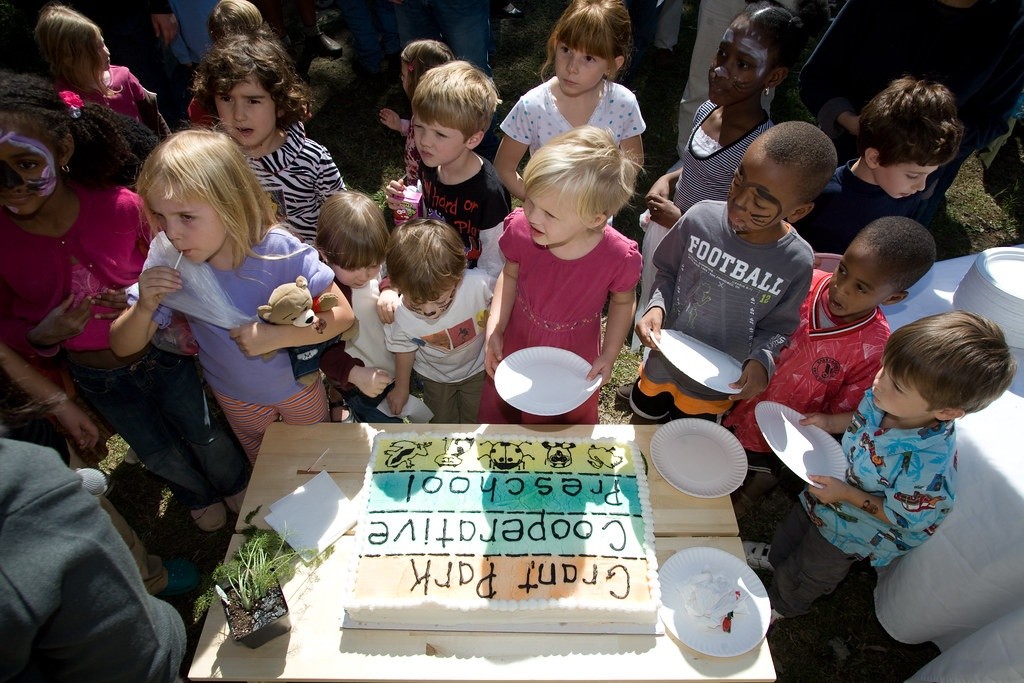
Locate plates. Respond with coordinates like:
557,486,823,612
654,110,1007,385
650,328,744,394
755,401,847,488
953,247,1024,350
650,418,748,498
494,346,601,415
658,547,771,657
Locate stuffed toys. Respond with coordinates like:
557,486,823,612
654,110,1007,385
261,275,338,386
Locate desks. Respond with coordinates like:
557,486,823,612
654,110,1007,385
874,238,1024,683
187,421,776,683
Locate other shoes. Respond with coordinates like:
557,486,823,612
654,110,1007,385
310,31,342,57
225,487,247,514
157,559,199,595
505,7,524,18
190,502,227,532
616,382,634,402
643,46,675,104
770,607,785,626
744,540,777,572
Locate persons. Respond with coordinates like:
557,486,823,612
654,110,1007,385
384,61,512,271
108,129,357,469
784,78,963,254
796,0,1024,228
1,334,189,683
1,72,257,533
617,2,807,404
722,217,936,526
477,125,643,426
492,0,644,228
380,40,455,233
733,310,1017,638
0,0,501,152
631,122,837,424
609,1,850,85
298,190,491,424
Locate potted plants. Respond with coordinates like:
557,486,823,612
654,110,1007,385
198,533,313,645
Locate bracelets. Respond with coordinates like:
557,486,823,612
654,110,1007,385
38,393,69,416
